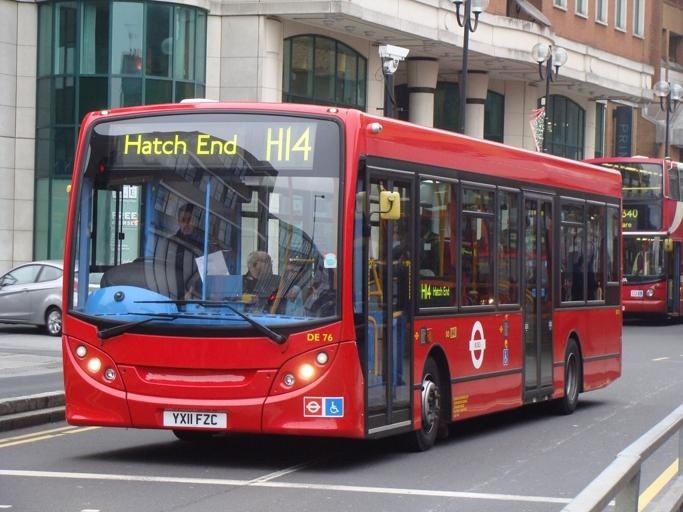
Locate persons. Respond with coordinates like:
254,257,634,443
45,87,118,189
176,202,203,242
420,221,451,278
243,251,304,314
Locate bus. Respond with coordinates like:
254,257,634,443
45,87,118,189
62,98,624,452
581,155,683,324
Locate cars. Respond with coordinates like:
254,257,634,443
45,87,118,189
0,259,100,337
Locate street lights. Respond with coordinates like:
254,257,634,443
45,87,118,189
653,79,683,158
531,41,568,153
452,0,489,135
